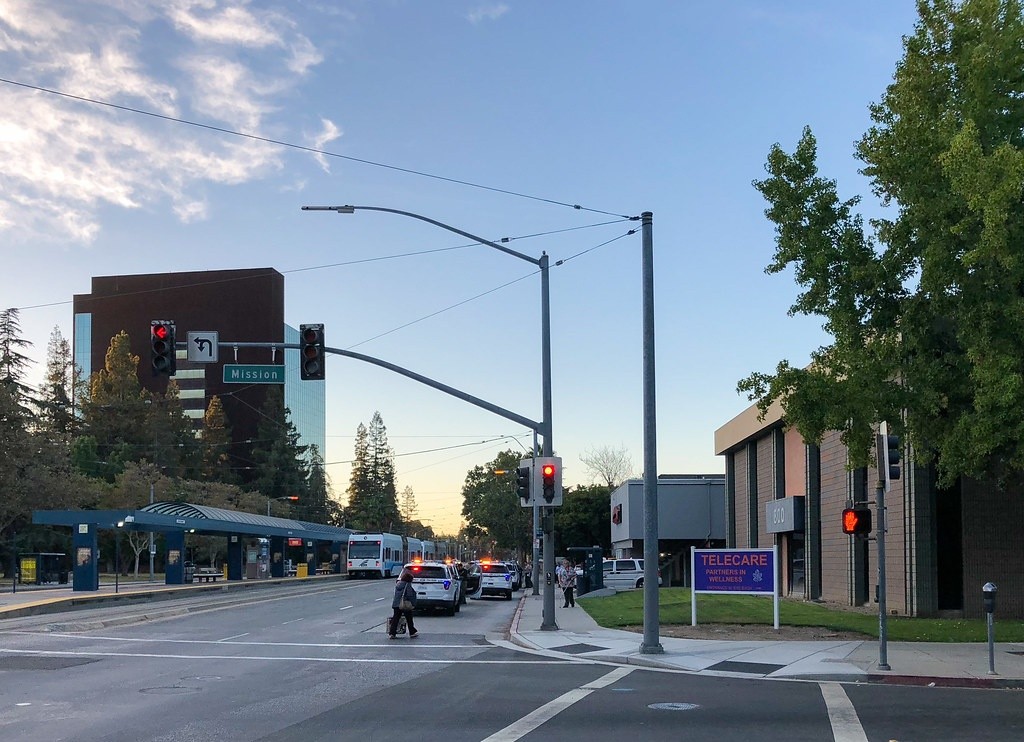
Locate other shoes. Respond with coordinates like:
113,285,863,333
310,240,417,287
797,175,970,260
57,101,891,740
572,603,574,607
390,634,396,639
563,605,568,608
410,632,419,638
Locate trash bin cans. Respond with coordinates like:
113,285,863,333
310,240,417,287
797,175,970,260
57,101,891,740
576,566,596,602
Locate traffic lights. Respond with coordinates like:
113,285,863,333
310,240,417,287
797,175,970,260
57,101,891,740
514,457,534,507
149,320,177,376
840,507,873,536
534,457,563,507
878,421,901,493
299,324,327,381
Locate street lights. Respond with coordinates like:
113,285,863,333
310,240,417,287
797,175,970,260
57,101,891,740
267,495,300,576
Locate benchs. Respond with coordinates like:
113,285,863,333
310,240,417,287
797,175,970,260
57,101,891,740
289,565,297,577
193,568,224,582
316,569,333,575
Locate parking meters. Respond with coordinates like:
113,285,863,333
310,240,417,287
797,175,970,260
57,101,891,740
981,581,1000,675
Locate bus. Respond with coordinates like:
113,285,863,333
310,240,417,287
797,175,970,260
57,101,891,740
346,531,435,579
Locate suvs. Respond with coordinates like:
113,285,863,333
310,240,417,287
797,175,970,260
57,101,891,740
396,558,483,616
573,557,663,589
467,559,523,601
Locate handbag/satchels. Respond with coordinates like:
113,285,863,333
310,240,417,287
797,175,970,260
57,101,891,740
386,615,406,634
399,582,415,610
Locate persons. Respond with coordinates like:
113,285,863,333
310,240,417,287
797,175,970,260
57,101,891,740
458,563,476,605
390,573,420,639
517,559,578,608
